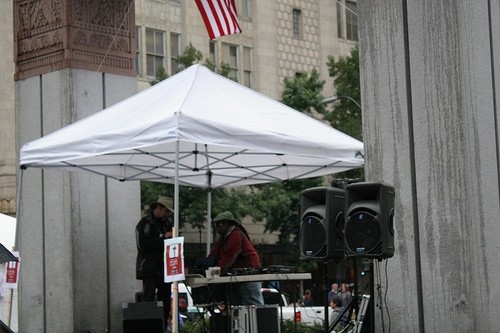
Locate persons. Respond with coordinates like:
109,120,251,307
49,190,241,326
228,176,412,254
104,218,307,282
328,283,338,308
304,289,313,306
135,196,174,333
207,211,264,305
330,295,346,333
338,283,351,306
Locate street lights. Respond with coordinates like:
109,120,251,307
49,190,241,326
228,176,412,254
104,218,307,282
320,94,362,112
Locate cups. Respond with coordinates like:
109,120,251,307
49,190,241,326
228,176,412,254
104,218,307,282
213,267,220,277
206,270,209,278
208,267,214,277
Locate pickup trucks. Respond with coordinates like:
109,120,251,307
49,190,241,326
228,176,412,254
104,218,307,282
259,288,333,325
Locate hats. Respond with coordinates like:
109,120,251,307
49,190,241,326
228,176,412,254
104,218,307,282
213,211,235,222
149,196,174,213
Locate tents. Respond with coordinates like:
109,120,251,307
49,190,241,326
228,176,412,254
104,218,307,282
8,65,364,333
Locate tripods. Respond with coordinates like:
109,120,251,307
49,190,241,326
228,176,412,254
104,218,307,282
328,258,363,333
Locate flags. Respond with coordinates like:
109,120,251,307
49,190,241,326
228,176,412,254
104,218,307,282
195,0,242,40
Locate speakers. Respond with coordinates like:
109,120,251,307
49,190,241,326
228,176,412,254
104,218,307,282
121,301,165,333
299,187,346,263
342,182,395,260
